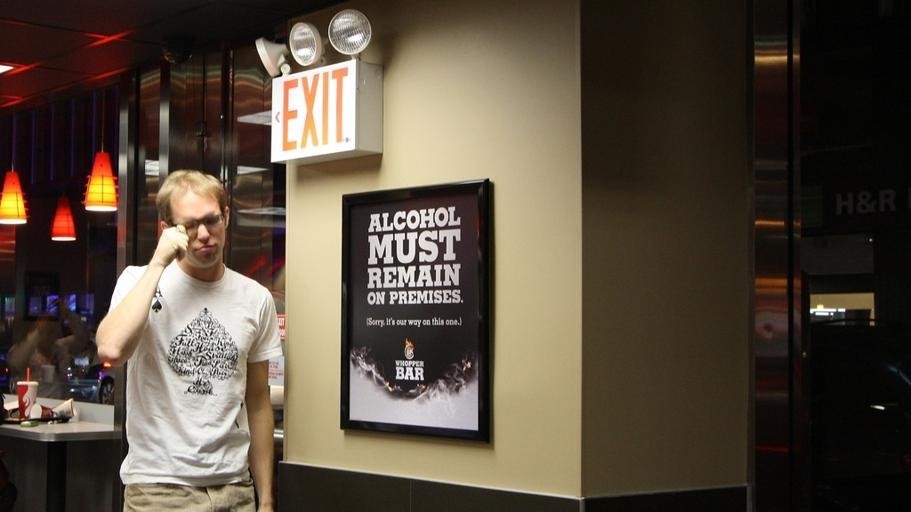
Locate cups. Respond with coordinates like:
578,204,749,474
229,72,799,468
39,365,56,384
16,381,75,420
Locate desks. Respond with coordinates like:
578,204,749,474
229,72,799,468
1,392,114,511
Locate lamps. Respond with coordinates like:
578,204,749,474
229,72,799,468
253,8,391,165
0,88,120,243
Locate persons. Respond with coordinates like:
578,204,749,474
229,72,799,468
5,296,90,399
93,168,282,512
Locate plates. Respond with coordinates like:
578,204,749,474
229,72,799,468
4,410,72,424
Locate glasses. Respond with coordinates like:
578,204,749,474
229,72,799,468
171,212,224,233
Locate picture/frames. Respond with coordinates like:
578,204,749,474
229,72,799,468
340,175,492,445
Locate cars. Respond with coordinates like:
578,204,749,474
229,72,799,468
66,346,114,403
